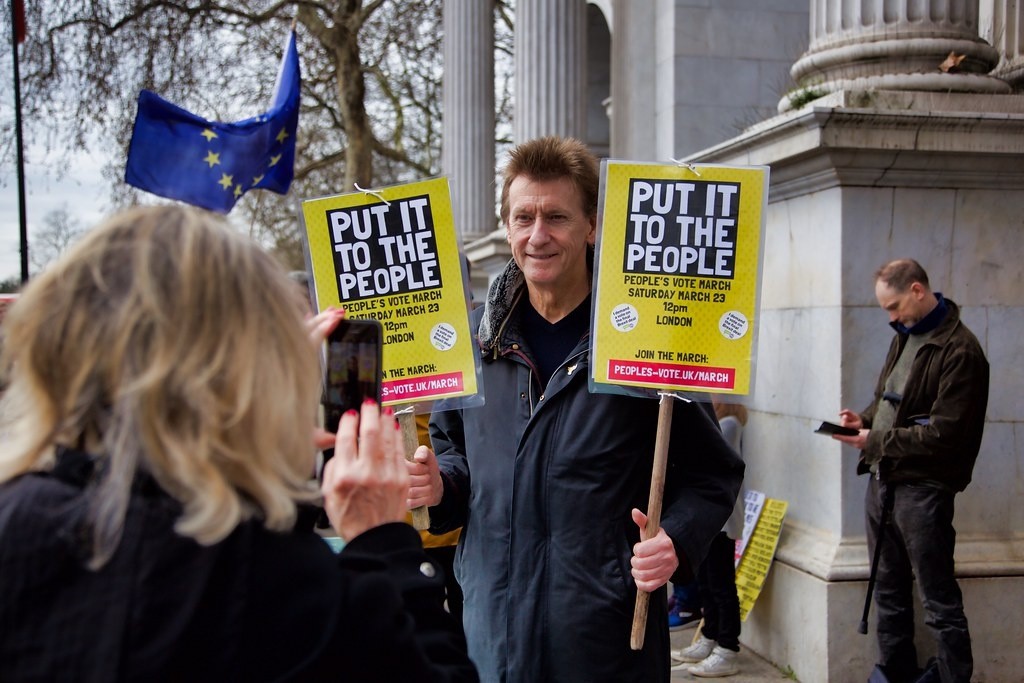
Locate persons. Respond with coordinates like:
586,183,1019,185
287,136,749,683
0,201,479,683
830,258,989,683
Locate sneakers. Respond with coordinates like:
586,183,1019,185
671,635,718,663
688,646,739,678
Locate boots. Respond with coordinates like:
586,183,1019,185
667,582,703,631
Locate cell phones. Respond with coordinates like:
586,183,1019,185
326,318,384,433
813,421,860,437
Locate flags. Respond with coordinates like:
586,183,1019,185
124,31,300,214
